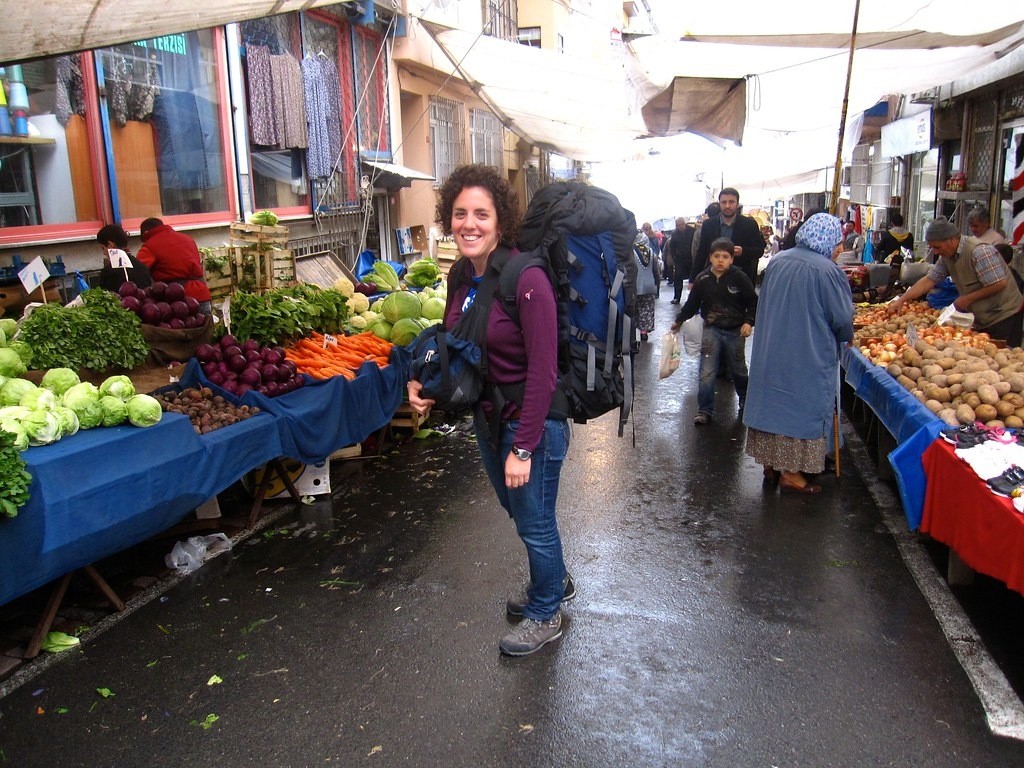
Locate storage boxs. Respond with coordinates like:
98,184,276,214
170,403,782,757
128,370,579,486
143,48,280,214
397,224,428,256
256,452,331,499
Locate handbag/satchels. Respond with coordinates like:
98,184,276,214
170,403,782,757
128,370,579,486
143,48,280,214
409,323,484,426
660,330,680,380
680,316,705,356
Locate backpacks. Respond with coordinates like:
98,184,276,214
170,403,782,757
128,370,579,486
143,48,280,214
498,182,638,449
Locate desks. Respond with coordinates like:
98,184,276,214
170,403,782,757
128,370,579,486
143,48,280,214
0,341,405,659
837,322,1024,591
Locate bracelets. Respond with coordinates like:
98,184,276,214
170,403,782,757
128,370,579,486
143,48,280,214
407,379,415,388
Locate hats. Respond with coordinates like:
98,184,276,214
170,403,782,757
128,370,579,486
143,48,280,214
925,215,958,242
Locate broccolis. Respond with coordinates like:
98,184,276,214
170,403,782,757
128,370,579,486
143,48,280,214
333,278,377,328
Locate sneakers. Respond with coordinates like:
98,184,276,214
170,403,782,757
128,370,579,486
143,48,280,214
737,403,745,414
507,571,577,615
693,410,717,424
500,607,561,657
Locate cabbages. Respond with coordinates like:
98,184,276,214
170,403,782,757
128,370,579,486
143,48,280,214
363,257,447,302
361,290,447,346
0,319,162,451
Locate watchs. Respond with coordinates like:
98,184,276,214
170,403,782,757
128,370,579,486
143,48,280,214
512,445,533,461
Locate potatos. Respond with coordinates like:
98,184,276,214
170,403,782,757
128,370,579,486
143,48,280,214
854,315,937,338
152,387,261,435
886,338,1024,429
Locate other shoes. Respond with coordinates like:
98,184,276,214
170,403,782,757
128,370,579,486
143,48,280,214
641,333,648,341
670,299,680,304
779,475,821,497
763,464,780,476
715,368,733,380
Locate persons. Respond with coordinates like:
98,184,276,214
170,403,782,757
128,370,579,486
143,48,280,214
406,162,639,655
136,218,212,318
839,220,863,263
97,224,151,294
632,187,767,424
889,206,1024,348
875,213,914,265
742,207,855,496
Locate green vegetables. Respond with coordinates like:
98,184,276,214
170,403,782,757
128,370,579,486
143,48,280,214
198,211,294,294
0,421,32,518
17,287,150,372
214,280,350,344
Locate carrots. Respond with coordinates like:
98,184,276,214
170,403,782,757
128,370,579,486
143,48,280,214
284,330,394,381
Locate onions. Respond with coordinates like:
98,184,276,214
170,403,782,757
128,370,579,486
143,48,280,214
354,282,369,293
197,335,305,397
860,327,997,367
854,301,942,324
367,282,377,290
118,282,206,328
